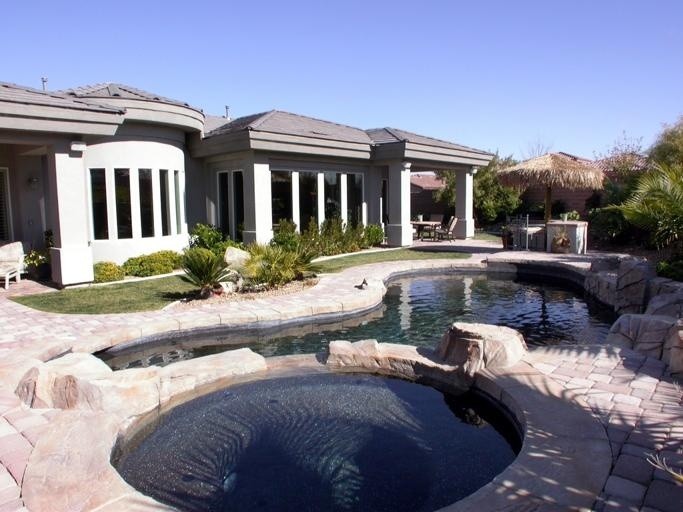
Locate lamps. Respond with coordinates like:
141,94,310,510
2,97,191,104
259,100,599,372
29,176,40,190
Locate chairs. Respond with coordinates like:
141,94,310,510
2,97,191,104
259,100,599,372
0,241,25,289
423,214,459,243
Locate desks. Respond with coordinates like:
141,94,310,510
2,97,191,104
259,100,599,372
410,221,442,241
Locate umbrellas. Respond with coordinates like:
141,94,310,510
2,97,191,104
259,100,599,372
494,152,604,252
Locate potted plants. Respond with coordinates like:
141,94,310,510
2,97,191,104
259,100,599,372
500,226,513,251
